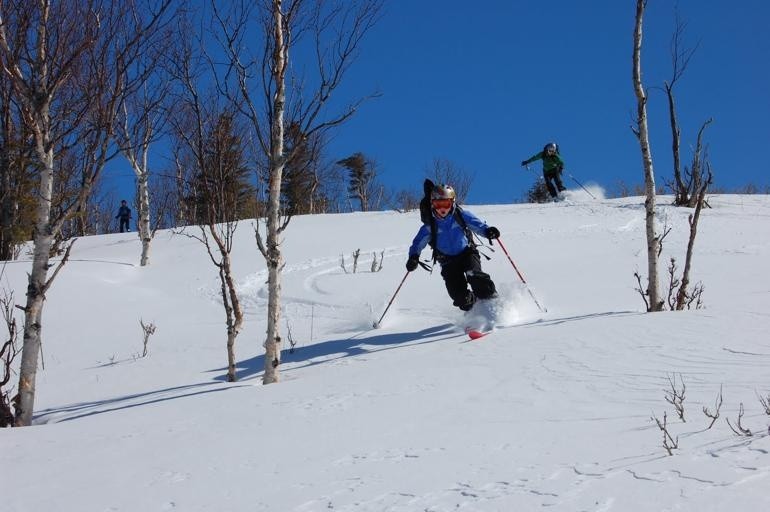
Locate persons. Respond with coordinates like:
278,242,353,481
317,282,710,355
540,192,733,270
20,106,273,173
406,184,500,340
115,200,132,233
521,143,567,202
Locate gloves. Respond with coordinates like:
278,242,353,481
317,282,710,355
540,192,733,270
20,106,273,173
522,161,528,165
406,254,420,271
486,227,500,239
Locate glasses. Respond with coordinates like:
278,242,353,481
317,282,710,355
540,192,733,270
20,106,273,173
433,200,453,209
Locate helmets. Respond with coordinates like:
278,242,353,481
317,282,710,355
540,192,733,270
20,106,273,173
429,183,455,202
547,143,556,156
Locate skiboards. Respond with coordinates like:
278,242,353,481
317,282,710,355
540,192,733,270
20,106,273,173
465,320,494,338
